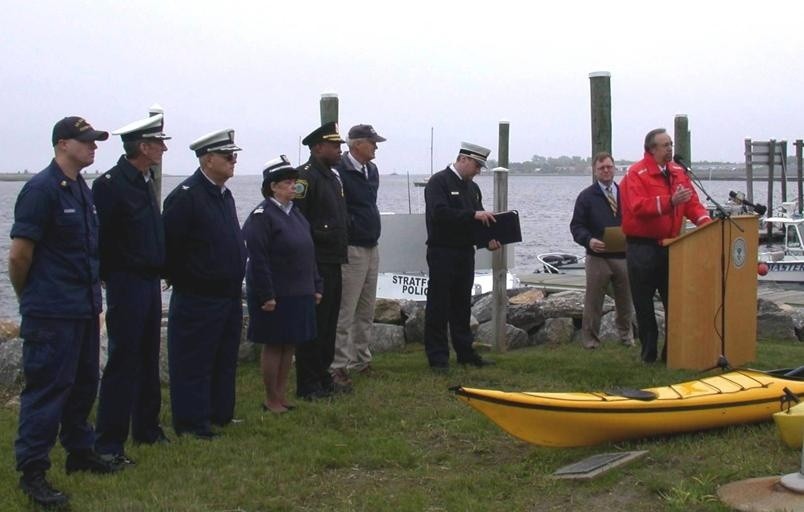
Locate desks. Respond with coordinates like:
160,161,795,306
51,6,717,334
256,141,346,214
766,215,804,254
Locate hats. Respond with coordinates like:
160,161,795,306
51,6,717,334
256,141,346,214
112,114,171,140
52,117,108,145
302,122,346,145
459,141,492,169
190,129,243,158
263,155,299,178
348,124,387,142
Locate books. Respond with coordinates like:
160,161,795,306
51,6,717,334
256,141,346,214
473,210,522,251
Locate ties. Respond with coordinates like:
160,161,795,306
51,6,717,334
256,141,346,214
606,187,618,218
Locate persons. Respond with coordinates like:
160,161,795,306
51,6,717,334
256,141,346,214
240,153,324,416
6,114,122,512
423,140,503,370
327,122,387,395
569,150,637,350
162,127,247,440
91,115,172,443
617,127,713,363
292,122,355,402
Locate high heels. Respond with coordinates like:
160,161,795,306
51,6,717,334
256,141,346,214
262,404,289,416
281,403,297,410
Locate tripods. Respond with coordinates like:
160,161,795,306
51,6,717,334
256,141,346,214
699,218,737,372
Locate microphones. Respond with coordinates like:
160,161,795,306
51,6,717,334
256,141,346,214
729,190,752,208
674,154,692,173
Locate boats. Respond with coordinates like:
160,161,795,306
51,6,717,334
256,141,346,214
450,367,804,448
773,398,804,448
376,170,521,301
535,201,804,284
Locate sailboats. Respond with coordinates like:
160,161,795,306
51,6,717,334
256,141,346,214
413,127,443,187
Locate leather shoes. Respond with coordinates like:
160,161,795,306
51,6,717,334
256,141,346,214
136,427,173,445
98,450,136,467
19,475,69,508
178,427,220,441
66,451,124,472
225,417,244,426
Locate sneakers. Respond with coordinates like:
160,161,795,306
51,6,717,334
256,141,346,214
623,339,635,346
457,355,494,368
431,365,450,377
297,365,389,404
583,339,600,349
641,351,658,363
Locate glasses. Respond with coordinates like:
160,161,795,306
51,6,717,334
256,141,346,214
597,166,613,170
